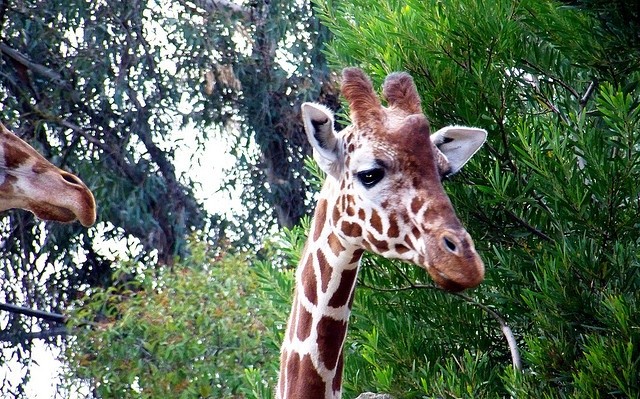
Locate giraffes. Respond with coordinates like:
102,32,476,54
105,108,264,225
273,67,488,399
0,121,97,228
